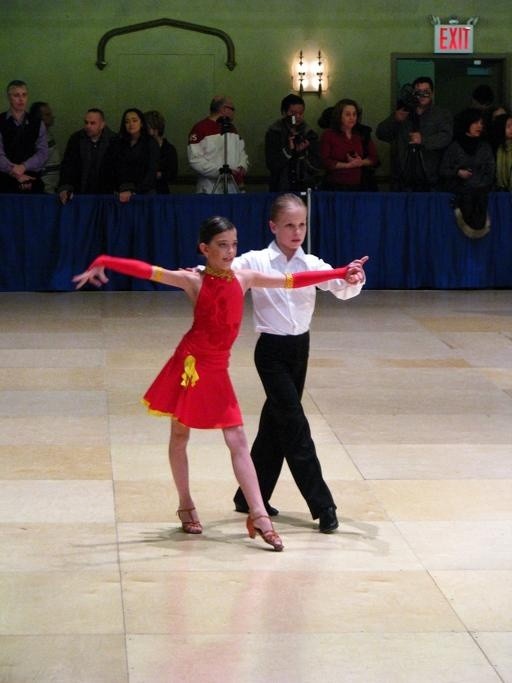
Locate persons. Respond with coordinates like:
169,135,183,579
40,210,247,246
177,192,367,534
70,215,365,553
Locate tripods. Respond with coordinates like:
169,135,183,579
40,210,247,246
396,114,429,192
211,137,241,194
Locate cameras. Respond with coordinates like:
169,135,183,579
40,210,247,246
216,115,238,136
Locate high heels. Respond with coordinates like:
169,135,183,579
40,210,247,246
178,506,202,533
247,515,283,551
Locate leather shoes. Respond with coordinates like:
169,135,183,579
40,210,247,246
235,498,278,515
319,506,338,532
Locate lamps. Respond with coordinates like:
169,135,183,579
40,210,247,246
293,49,325,94
